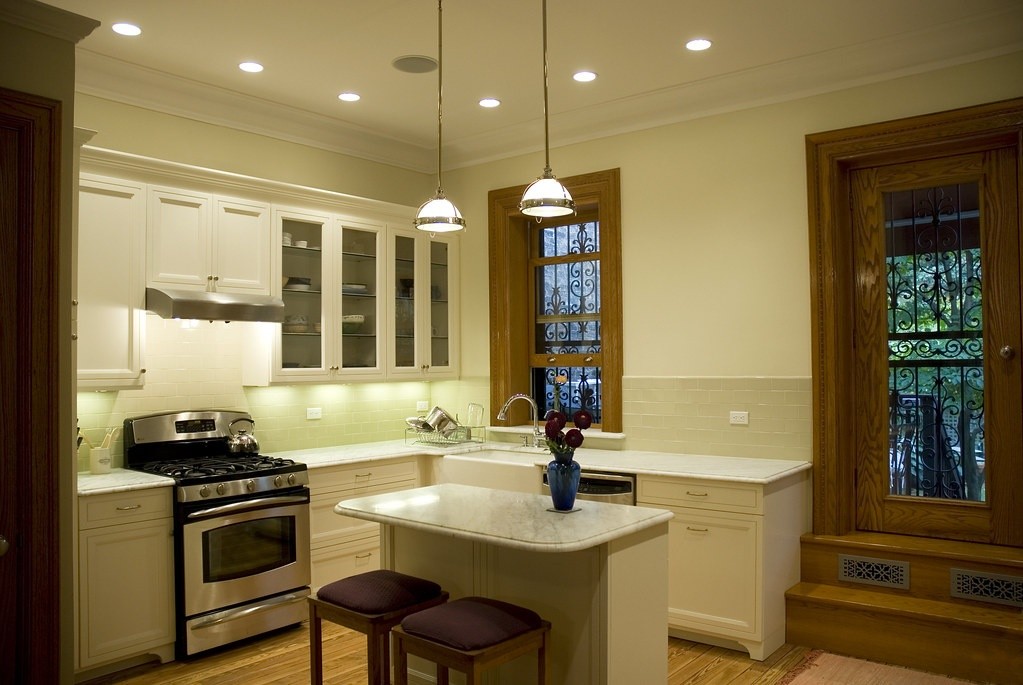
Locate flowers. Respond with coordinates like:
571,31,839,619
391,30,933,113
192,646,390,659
544,411,592,452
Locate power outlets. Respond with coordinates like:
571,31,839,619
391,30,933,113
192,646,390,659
417,400,428,411
729,411,749,424
307,408,322,420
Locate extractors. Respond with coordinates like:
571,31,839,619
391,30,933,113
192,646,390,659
145,287,285,323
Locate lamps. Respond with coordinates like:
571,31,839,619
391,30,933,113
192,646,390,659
516,0,576,224
413,0,466,237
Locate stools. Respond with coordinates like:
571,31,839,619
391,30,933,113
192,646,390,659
306,570,449,685
388,596,551,685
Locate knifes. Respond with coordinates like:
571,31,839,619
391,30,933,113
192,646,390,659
76,419,83,450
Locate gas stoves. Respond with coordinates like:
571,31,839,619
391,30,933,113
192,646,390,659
123,409,309,502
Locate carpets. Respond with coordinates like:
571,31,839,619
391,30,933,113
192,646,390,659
776,650,970,685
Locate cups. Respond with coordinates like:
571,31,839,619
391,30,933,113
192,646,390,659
402,287,414,297
282,231,292,246
431,286,440,300
295,241,307,248
431,326,438,336
89,447,111,475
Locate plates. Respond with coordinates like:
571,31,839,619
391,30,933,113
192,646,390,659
303,365,320,367
406,417,425,429
342,288,367,293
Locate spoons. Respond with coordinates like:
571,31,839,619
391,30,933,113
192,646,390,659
107,427,119,447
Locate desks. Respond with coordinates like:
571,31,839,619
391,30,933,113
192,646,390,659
334,482,676,685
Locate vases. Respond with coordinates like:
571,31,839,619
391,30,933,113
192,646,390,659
547,450,580,511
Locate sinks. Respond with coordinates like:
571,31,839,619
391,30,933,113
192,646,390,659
443,450,549,468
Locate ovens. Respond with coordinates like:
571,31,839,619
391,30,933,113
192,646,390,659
175,487,311,663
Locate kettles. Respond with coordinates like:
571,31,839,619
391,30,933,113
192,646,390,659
226,418,260,457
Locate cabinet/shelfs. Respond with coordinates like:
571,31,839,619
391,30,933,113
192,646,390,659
635,474,813,661
73,489,176,685
72,146,457,387
308,455,428,594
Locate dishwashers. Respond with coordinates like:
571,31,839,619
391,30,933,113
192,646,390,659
541,465,635,506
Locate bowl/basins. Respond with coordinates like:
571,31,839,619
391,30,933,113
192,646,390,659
282,363,299,368
400,278,414,287
343,283,367,289
426,407,458,440
313,323,321,332
342,314,364,334
287,277,312,290
283,314,309,332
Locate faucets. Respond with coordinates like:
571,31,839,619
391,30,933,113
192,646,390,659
496,393,546,448
